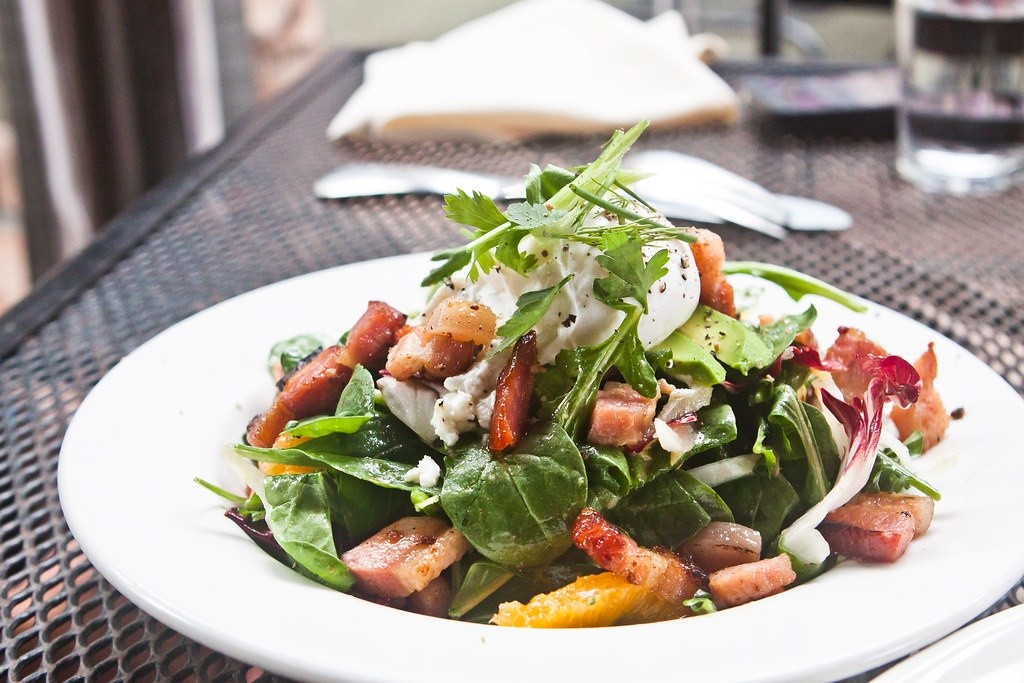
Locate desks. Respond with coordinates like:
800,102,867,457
0,48,1024,683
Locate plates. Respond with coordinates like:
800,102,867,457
57,250,1024,683
868,603,1024,683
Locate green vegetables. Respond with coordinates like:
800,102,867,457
191,117,943,618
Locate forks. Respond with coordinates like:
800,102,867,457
312,150,787,241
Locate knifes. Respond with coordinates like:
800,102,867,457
626,193,854,230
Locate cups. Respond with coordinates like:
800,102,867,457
895,0,1024,192
